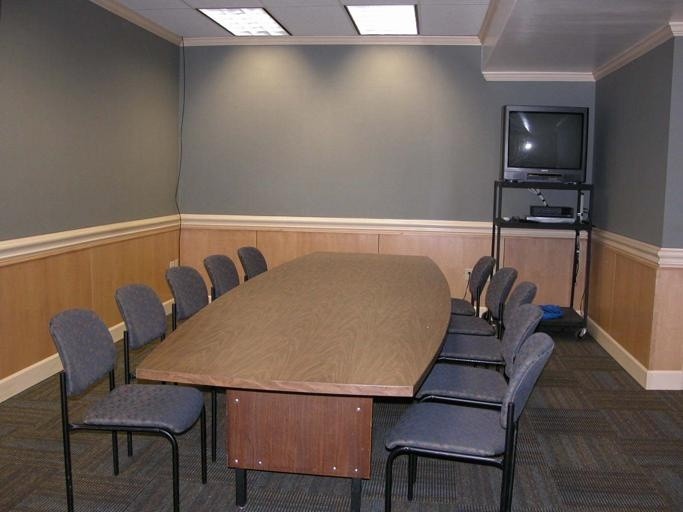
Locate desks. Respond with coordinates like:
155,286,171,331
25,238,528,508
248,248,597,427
136,252,453,512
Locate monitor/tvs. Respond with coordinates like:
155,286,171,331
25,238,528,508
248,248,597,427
501,103,590,185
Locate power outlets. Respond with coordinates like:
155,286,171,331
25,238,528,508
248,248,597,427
464,268,473,281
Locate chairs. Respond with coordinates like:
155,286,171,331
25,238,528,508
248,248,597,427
437,281,537,369
204,254,240,302
165,266,209,330
449,257,495,315
237,246,266,282
383,332,554,511
413,302,544,411
50,307,207,511
447,267,518,338
115,283,218,461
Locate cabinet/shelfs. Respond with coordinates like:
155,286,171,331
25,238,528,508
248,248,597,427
490,180,595,340
